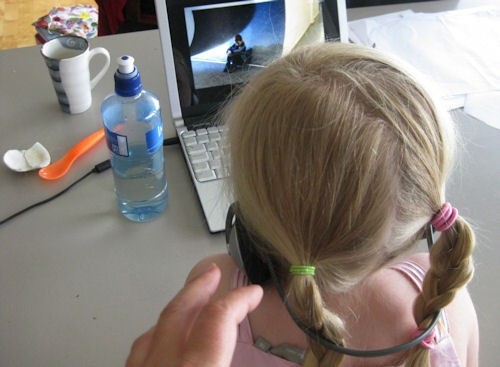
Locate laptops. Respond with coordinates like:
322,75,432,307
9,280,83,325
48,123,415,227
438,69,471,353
152,0,350,237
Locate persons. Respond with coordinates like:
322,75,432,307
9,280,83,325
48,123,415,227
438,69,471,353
222,34,247,72
185,42,480,366
123,260,265,367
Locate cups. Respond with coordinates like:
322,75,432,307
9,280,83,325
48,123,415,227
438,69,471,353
40,35,111,115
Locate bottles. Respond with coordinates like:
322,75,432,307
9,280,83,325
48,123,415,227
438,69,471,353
101,55,167,223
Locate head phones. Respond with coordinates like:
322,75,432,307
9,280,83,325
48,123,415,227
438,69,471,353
224,203,446,358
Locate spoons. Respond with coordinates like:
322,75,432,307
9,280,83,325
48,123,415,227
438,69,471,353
39,129,106,179
